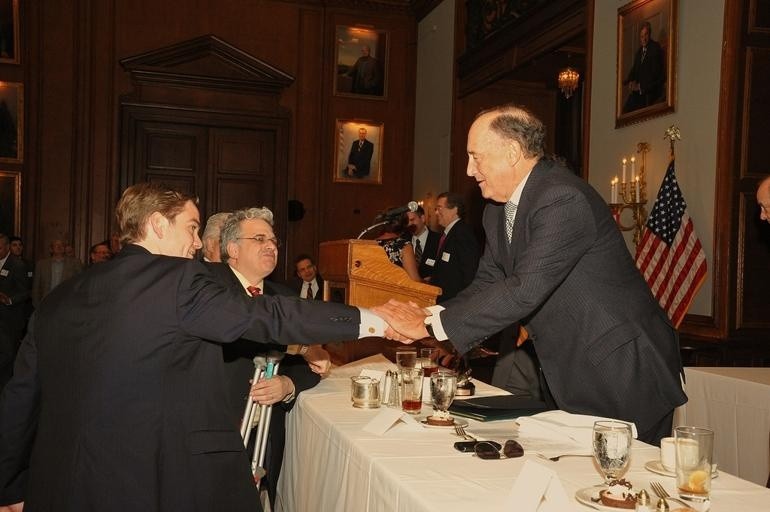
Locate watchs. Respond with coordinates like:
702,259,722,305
424,315,435,337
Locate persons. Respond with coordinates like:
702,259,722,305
371,103,689,447
207,208,332,512
33,238,81,311
340,44,380,95
200,213,230,263
2,235,34,387
89,243,112,264
64,245,74,257
621,21,664,114
376,207,424,283
429,192,471,301
340,128,373,179
405,206,440,280
755,175,770,227
288,255,343,304
2,179,432,512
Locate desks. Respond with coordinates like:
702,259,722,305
679,360,770,488
273,351,770,512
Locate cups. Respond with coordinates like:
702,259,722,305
430,370,457,414
400,367,425,413
673,425,714,512
421,347,440,376
350,375,381,409
592,419,633,480
396,347,417,370
660,437,702,471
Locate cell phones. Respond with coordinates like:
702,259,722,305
455,441,503,452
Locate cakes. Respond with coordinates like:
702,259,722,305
600,479,638,509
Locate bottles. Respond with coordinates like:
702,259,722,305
382,369,399,409
634,489,669,511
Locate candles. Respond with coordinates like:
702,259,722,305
607,154,641,203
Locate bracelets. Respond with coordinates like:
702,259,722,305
300,344,309,355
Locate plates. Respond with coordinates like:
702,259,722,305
645,460,719,479
575,485,661,510
415,416,468,428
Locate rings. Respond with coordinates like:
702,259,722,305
270,394,273,400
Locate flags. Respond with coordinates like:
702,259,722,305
634,159,708,330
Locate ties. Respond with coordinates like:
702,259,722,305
414,240,423,266
307,283,313,300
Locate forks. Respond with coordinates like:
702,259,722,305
454,423,475,439
537,454,594,461
648,480,696,510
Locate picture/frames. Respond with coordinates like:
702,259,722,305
331,18,391,103
612,0,681,132
0,79,26,166
0,170,24,241
333,115,386,187
0,0,21,64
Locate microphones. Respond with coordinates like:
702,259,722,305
377,201,418,219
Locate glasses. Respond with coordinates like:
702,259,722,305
236,235,281,246
